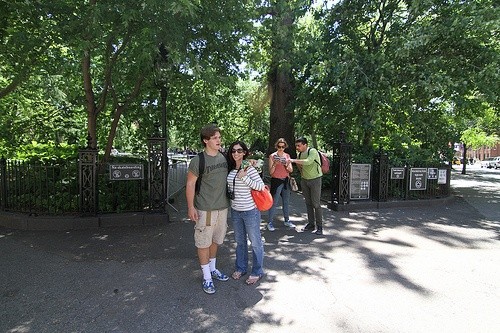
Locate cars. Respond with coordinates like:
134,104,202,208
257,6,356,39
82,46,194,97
444,155,476,166
480,156,500,169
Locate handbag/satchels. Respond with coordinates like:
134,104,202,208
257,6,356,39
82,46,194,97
289,177,299,191
244,166,273,211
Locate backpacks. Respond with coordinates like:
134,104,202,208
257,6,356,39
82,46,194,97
299,147,330,174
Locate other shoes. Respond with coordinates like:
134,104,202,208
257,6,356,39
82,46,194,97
232,271,247,280
245,274,264,285
267,221,275,231
210,269,230,281
315,226,322,235
202,278,215,294
284,221,296,228
304,224,315,230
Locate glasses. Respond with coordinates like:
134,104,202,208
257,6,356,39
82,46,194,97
295,145,302,147
232,149,244,153
277,145,285,148
210,136,222,140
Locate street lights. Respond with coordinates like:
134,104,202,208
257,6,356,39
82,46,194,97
154,62,175,211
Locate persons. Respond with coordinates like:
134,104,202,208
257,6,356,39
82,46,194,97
289,138,323,235
267,138,296,231
246,150,264,173
186,126,229,293
220,145,227,155
227,141,265,284
